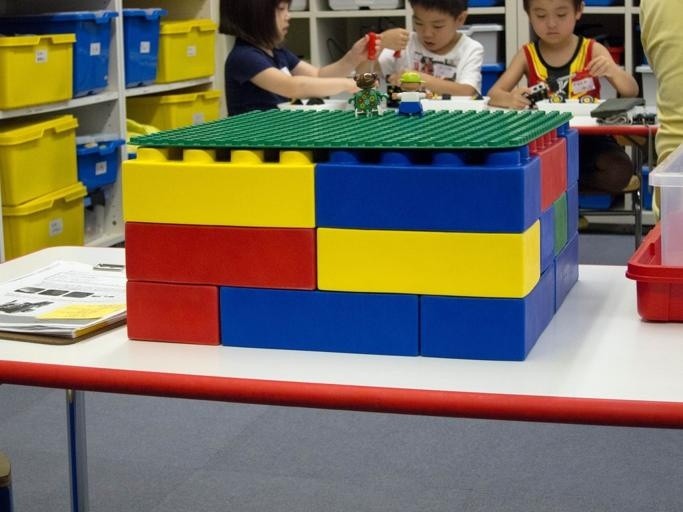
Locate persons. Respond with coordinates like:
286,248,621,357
218,1,381,118
632,0,683,217
355,0,483,99
488,1,640,231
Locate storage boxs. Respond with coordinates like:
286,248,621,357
120,6,168,91
276,0,683,322
76,135,125,194
1,113,79,211
123,89,225,136
160,17,217,85
0,30,76,109
44,11,119,96
1,183,90,261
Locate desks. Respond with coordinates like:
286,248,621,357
0,245,682,512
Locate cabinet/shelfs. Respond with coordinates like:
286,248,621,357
0,0,226,250
